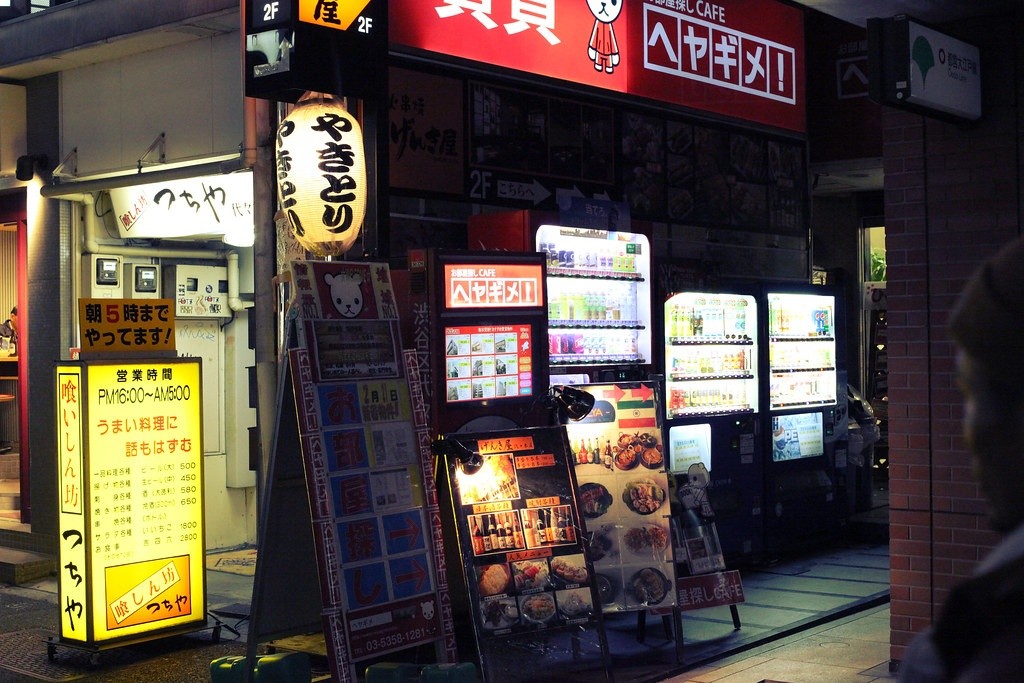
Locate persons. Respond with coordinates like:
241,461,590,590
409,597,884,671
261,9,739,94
893,230,1024,682
1,305,18,352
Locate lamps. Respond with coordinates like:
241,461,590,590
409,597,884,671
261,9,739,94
542,386,595,427
431,437,484,476
15,154,49,181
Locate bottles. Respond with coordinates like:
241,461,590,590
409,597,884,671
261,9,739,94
670,385,748,409
672,348,746,377
468,511,525,555
568,434,613,470
668,303,747,339
523,506,576,548
582,287,606,321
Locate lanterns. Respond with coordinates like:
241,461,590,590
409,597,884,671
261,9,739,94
275,98,368,256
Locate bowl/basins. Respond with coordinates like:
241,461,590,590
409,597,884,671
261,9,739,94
520,592,556,625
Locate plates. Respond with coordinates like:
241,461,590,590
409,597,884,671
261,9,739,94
625,566,672,607
622,522,669,557
577,482,613,519
